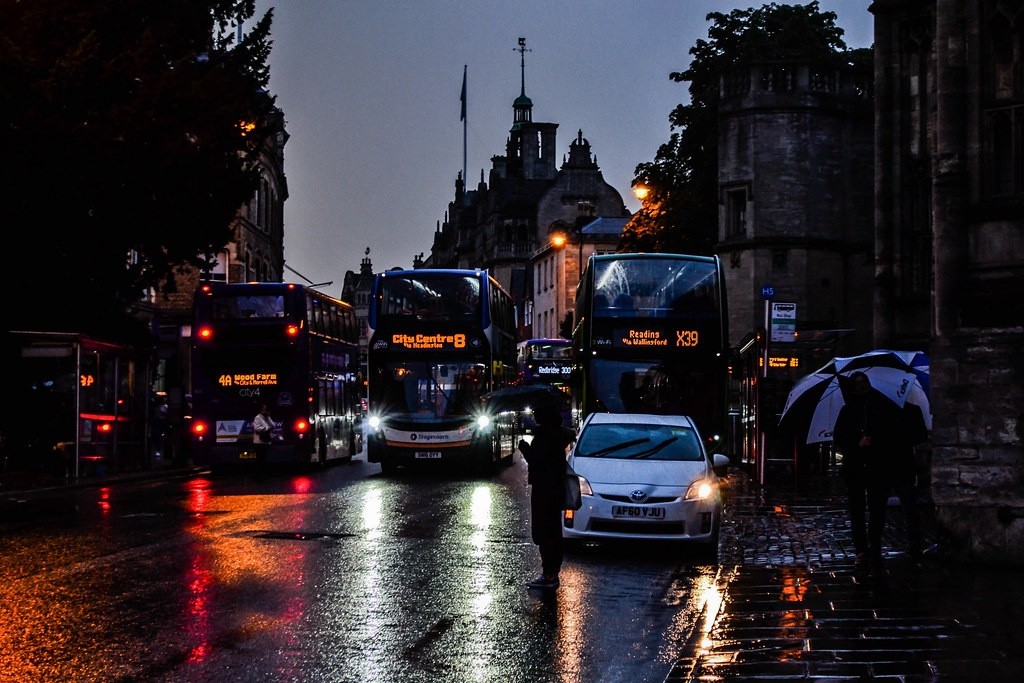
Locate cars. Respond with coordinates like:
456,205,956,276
561,412,730,556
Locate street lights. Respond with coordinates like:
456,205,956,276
551,230,585,284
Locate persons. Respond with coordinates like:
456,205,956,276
834,372,929,568
519,397,576,589
253,404,277,484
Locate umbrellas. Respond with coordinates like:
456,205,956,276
486,384,569,412
778,348,934,446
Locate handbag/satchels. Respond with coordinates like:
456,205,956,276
565,459,595,511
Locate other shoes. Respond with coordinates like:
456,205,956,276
854,556,866,566
526,577,560,588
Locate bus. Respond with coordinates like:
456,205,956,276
185,280,361,475
361,266,522,480
514,338,579,434
572,251,742,469
